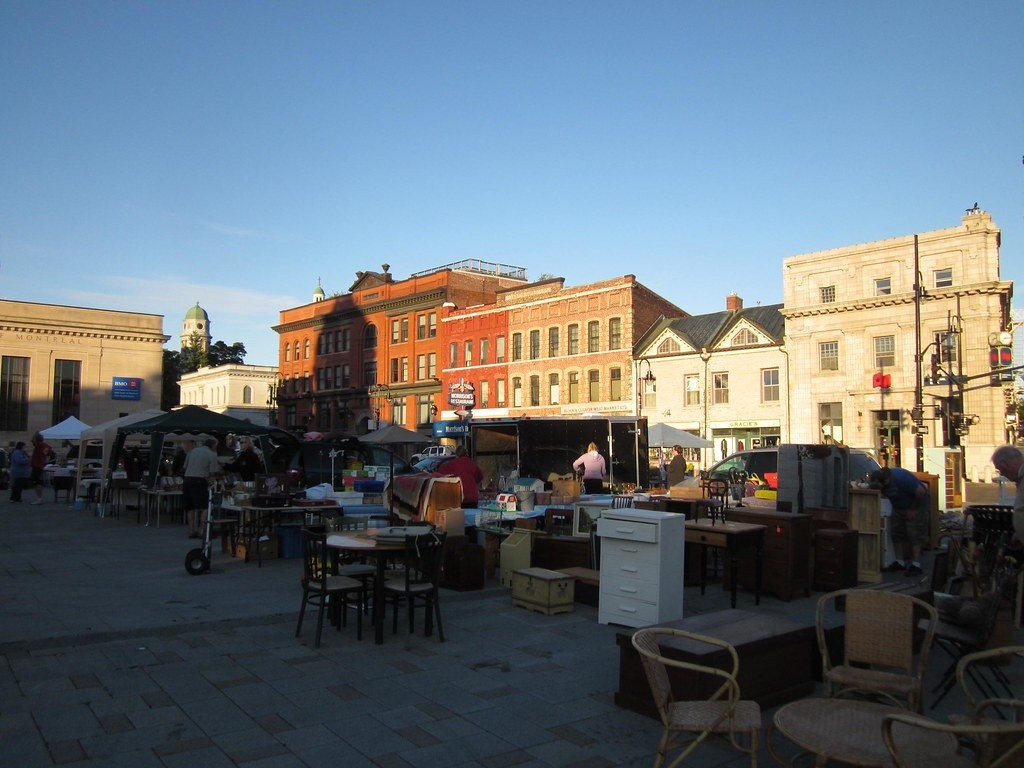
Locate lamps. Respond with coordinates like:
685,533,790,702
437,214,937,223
308,413,314,421
346,410,354,419
430,405,437,415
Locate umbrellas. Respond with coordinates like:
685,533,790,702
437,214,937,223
357,426,432,445
648,423,713,479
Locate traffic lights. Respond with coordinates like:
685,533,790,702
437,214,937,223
999,346,1013,367
990,346,999,368
931,353,942,380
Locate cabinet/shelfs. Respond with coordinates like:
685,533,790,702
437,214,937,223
443,534,484,593
811,526,860,593
508,568,577,617
725,506,814,602
475,527,591,582
613,606,816,727
597,510,685,627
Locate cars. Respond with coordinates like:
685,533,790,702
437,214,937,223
407,445,457,472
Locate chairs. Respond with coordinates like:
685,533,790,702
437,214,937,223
50,476,1023,768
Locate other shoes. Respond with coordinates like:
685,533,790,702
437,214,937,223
31,499,42,505
199,534,205,540
189,533,198,539
8,499,14,502
15,499,24,502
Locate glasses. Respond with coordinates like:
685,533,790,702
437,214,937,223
240,442,246,444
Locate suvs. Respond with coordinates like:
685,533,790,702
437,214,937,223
266,441,417,492
42,440,187,490
665,444,893,532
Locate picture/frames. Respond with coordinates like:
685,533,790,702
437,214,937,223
572,503,609,538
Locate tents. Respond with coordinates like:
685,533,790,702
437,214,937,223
41,415,91,440
75,409,168,518
101,406,269,527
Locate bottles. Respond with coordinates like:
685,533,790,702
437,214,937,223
498,475,506,493
864,471,870,483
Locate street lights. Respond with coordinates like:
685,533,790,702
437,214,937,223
273,372,289,427
634,358,657,488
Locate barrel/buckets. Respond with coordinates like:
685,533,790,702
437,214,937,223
516,491,535,512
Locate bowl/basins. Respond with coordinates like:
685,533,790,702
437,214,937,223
860,483,869,489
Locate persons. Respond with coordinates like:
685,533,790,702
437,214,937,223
438,446,482,543
664,445,686,487
870,467,929,576
573,442,606,494
220,436,262,480
992,447,1024,659
181,439,221,540
8,442,31,503
30,436,50,505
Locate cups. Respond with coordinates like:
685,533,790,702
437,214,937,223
611,482,636,495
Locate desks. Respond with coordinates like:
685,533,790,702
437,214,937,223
81,479,101,511
765,696,958,767
317,529,430,644
145,490,183,529
109,483,154,521
685,518,767,607
224,505,343,567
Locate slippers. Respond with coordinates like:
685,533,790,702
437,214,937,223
904,564,923,577
887,561,905,572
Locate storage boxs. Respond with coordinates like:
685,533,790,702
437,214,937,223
235,464,391,562
495,493,516,511
